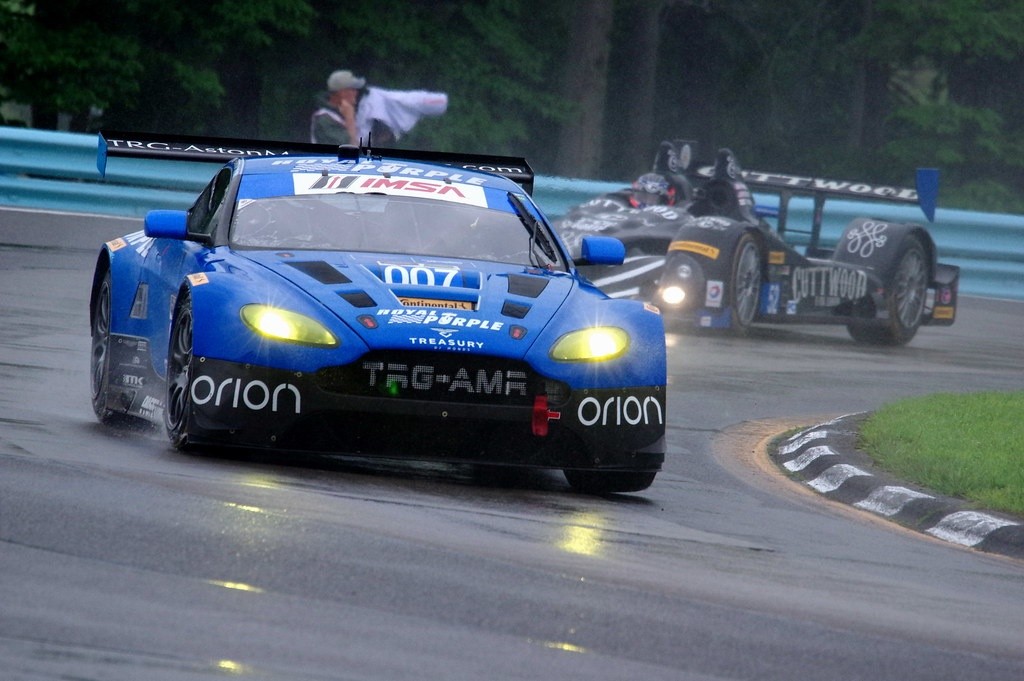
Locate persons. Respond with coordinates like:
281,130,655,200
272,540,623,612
310,70,392,147
628,172,676,210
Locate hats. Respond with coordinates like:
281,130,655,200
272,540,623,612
326,70,365,94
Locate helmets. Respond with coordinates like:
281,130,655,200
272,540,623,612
630,172,675,209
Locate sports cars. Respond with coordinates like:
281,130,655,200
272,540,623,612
87,138,670,496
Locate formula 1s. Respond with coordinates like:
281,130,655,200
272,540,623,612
547,139,963,348
